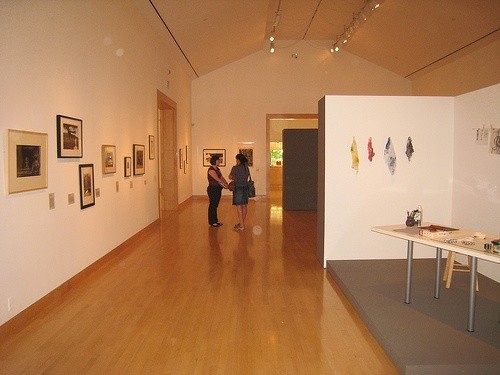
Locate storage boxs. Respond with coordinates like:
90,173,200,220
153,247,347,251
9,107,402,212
419,229,463,240
276,161,281,165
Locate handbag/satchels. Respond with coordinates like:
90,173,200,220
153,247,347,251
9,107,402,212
247,181,255,198
228,181,236,191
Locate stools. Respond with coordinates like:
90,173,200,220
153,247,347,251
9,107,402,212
443,251,479,291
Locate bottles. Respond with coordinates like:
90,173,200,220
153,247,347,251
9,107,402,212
406,205,423,227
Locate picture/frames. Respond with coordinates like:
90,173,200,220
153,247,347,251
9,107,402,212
5,129,49,194
101,144,116,175
148,135,156,160
124,157,131,177
239,149,253,167
179,145,189,175
57,115,83,159
79,164,95,210
203,149,226,167
133,144,145,175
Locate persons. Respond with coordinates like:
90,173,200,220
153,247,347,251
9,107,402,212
228,154,250,231
207,156,228,227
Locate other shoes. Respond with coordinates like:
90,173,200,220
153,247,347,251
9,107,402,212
233,224,244,232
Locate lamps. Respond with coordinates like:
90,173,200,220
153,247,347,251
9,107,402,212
330,0,383,53
269,11,281,54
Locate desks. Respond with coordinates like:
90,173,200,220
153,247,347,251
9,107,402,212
370,221,500,332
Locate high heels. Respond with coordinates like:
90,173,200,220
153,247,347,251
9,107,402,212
210,222,223,227
